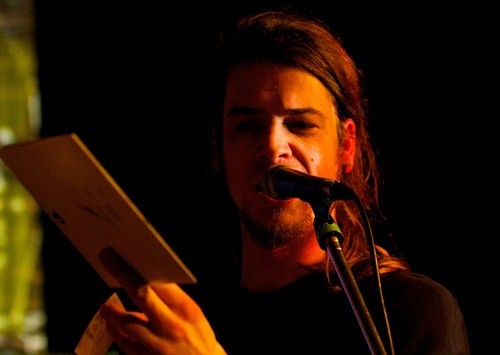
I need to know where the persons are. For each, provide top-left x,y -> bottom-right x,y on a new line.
98,12 -> 470,355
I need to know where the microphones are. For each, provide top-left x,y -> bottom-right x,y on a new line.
260,164 -> 353,202
